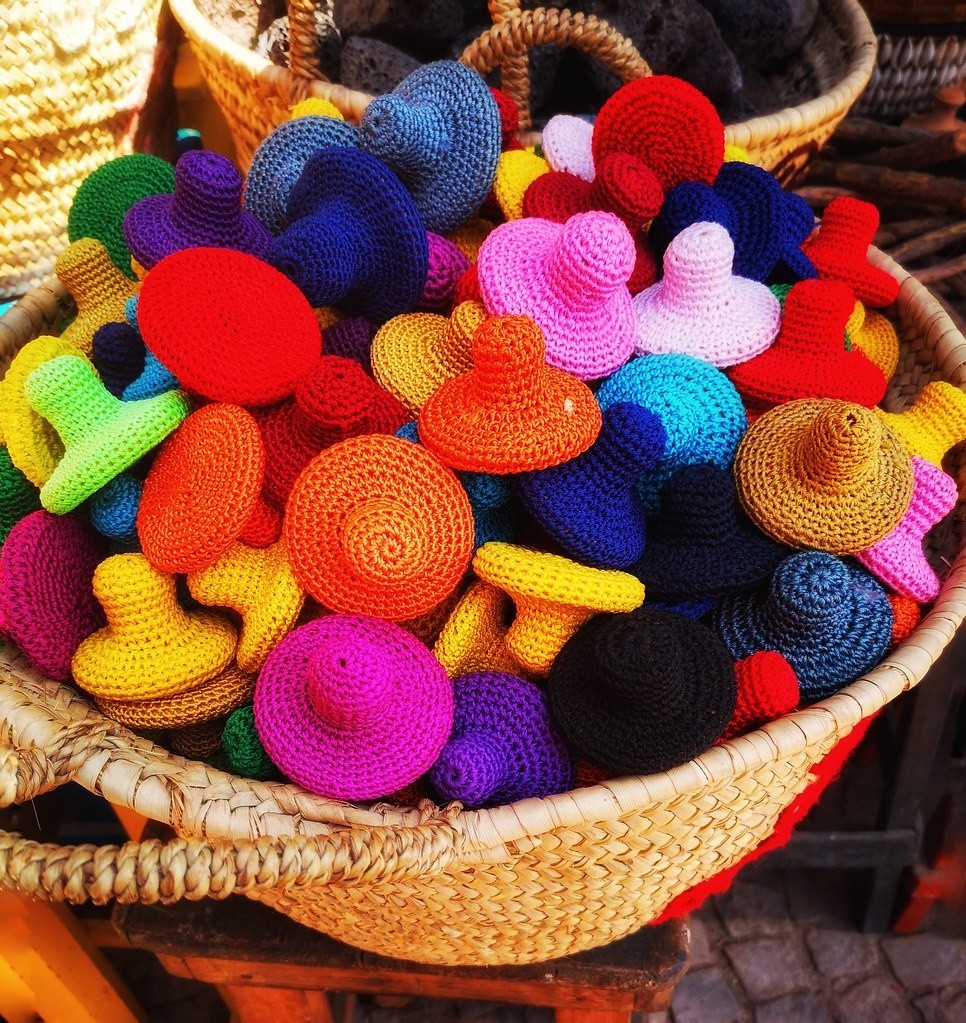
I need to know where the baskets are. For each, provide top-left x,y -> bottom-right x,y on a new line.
0,0 -> 966,968
